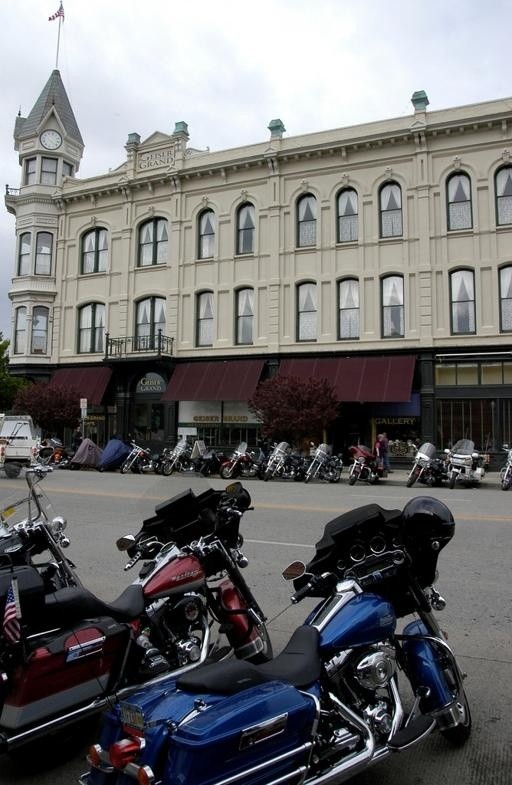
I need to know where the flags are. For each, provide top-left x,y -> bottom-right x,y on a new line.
3,578 -> 23,645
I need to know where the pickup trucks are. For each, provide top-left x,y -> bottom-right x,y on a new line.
0,414 -> 42,479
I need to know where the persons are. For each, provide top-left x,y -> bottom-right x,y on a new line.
72,427 -> 83,447
373,432 -> 384,476
381,432 -> 394,473
134,428 -> 145,442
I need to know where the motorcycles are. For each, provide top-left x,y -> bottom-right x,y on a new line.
500,444 -> 512,491
